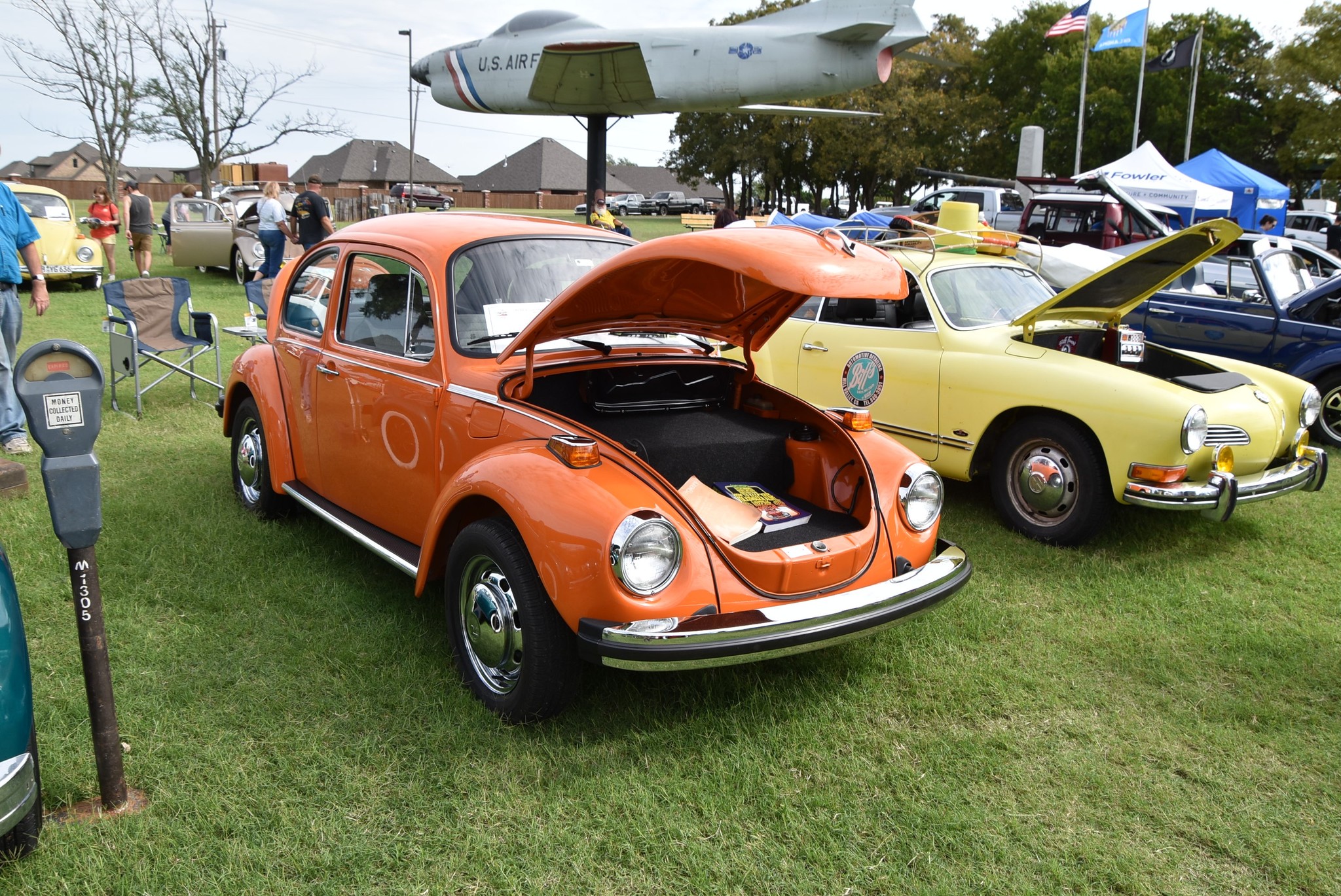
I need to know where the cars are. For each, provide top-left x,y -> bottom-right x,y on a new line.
1101,233 -> 1341,307
216,213 -> 973,730
169,179 -> 338,286
1012,176 -> 1189,250
389,183 -> 455,211
871,186 -> 1091,234
608,218 -> 1329,546
574,196 -> 616,216
1010,229 -> 1341,449
187,183 -> 227,213
1,180 -> 105,292
1283,210 -> 1338,251
605,193 -> 646,216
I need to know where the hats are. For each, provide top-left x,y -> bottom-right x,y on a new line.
124,180 -> 138,190
597,199 -> 605,204
308,175 -> 321,184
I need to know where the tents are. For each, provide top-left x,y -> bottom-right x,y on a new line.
1070,140 -> 1234,226
1161,147 -> 1291,237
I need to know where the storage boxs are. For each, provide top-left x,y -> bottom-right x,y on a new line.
217,161 -> 289,187
899,200 -> 1023,256
1287,198 -> 1337,213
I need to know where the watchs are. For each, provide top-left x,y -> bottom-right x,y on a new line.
32,274 -> 45,280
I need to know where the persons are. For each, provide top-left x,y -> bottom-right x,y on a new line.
756,196 -> 762,216
747,196 -> 755,216
714,208 -> 740,229
590,199 -> 633,238
122,180 -> 154,279
87,186 -> 121,281
1326,214 -> 1341,259
1258,214 -> 1277,234
0,182 -> 51,454
290,175 -> 335,253
252,181 -> 299,281
161,184 -> 197,257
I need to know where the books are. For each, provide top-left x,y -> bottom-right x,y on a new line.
711,481 -> 812,534
678,474 -> 763,546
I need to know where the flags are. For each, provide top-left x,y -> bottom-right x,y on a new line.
1145,34 -> 1196,73
1306,179 -> 1321,197
1044,0 -> 1091,39
1090,8 -> 1148,52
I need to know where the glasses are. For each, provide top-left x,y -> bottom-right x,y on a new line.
93,194 -> 104,199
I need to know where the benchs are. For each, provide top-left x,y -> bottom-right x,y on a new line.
791,298 -> 898,328
744,216 -> 770,226
681,213 -> 740,232
764,214 -> 793,219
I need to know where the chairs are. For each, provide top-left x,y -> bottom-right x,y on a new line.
101,277 -> 225,424
152,222 -> 168,255
836,298 -> 893,328
351,274 -> 435,354
1160,274 -> 1192,293
1181,262 -> 1218,295
898,289 -> 936,331
221,277 -> 276,347
1227,247 -> 1252,268
506,265 -> 593,303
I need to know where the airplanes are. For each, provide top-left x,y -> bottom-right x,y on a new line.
414,0 -> 968,118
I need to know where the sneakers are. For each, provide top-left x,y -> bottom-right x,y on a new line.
1,437 -> 33,454
139,270 -> 149,279
107,273 -> 115,281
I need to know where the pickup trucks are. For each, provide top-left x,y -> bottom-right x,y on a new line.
638,191 -> 704,217
759,195 -> 811,216
826,199 -> 871,218
